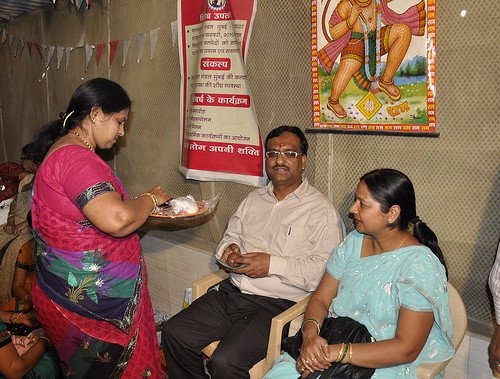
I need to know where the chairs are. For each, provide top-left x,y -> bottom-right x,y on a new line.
265,281 -> 467,379
191,270 -> 305,379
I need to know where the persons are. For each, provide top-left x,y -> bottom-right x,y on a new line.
163,125 -> 346,379
0,142 -> 48,312
487,241 -> 500,374
0,312 -> 53,379
264,168 -> 457,378
22,76 -> 174,379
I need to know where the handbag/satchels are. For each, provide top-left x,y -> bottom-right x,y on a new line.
291,318 -> 376,379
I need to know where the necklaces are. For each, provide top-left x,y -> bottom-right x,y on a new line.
395,234 -> 411,249
68,128 -> 95,153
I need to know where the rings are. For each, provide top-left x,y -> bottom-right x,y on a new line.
298,344 -> 328,372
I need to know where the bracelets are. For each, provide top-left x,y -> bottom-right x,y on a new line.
135,191 -> 159,215
37,336 -> 52,346
12,312 -> 24,324
349,342 -> 353,364
301,318 -> 321,335
9,312 -> 18,324
7,214 -> 15,219
335,343 -> 345,364
339,343 -> 348,364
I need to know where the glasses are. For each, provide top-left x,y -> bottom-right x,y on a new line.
265,150 -> 303,158
